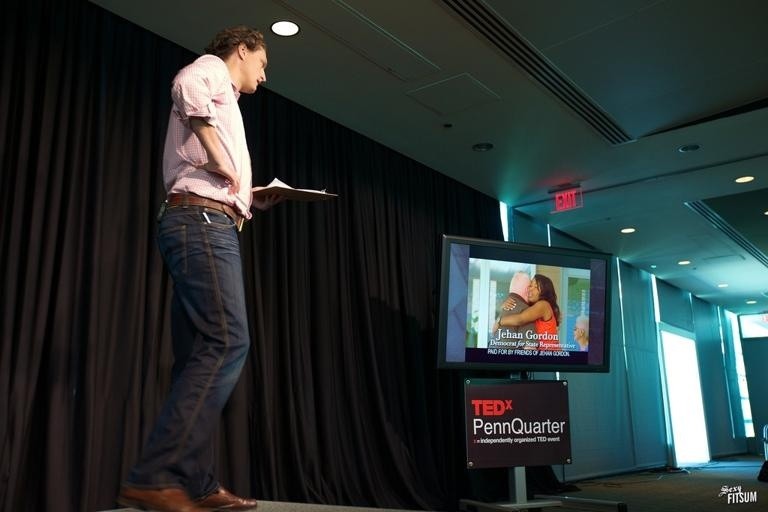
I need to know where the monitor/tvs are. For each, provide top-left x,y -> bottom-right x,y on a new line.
436,233 -> 611,372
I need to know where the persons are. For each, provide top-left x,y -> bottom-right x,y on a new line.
488,272 -> 539,351
116,23 -> 286,511
492,274 -> 562,352
573,316 -> 589,352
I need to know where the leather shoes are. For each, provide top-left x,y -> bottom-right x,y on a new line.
118,485 -> 257,512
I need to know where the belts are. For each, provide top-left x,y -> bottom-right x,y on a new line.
166,193 -> 244,232
